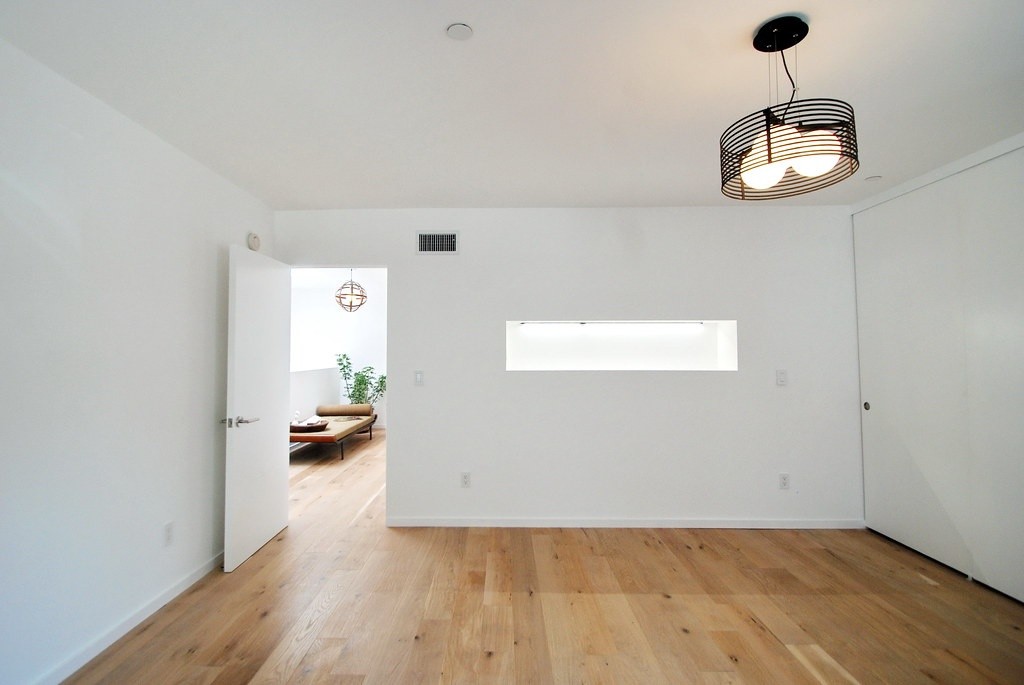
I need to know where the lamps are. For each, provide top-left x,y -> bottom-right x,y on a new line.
720,14 -> 860,202
335,268 -> 368,313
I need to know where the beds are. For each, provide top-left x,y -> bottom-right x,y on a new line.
290,412 -> 378,460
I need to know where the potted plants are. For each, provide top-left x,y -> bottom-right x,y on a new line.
337,353 -> 386,434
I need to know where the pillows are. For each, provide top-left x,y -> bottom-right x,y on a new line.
316,404 -> 374,417
